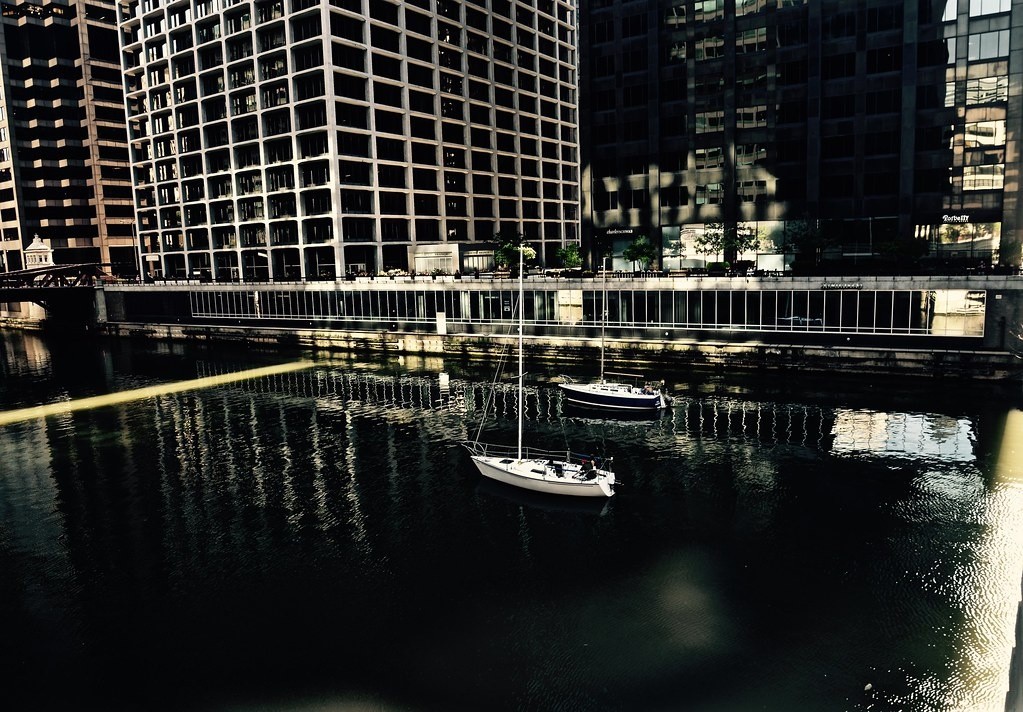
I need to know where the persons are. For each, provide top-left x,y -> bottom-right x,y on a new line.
977,260 -> 985,275
580,460 -> 597,481
434,269 -> 441,279
473,267 -> 479,279
410,269 -> 416,280
369,270 -> 374,280
662,266 -> 671,278
685,268 -> 692,277
455,270 -> 459,279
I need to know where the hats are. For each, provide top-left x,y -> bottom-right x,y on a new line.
580,459 -> 587,464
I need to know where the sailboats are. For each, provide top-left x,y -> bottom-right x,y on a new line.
460,232 -> 623,497
557,251 -> 692,410
